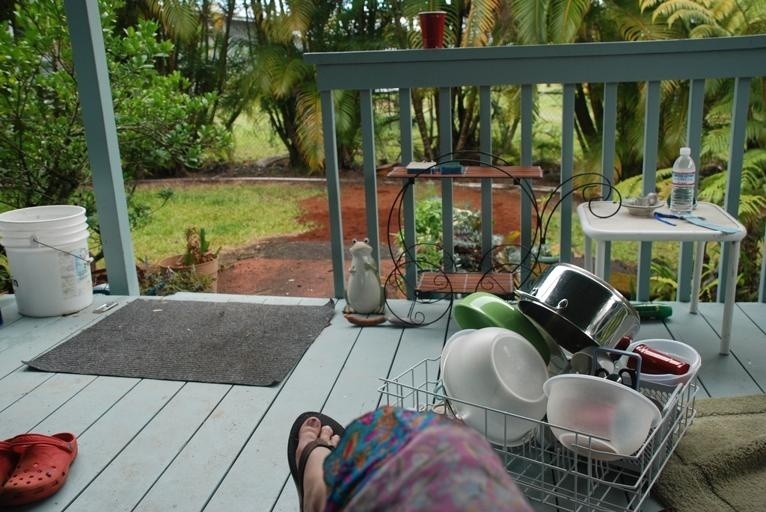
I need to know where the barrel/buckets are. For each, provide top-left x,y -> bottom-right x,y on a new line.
1,203 -> 95,318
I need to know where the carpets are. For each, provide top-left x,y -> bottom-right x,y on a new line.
639,391 -> 766,511
20,296 -> 337,388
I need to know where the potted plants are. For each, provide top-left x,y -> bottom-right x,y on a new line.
158,227 -> 224,294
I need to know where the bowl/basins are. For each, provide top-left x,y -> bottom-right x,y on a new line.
441,327 -> 551,451
617,339 -> 703,389
540,374 -> 663,463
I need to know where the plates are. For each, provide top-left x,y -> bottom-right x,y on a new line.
451,289 -> 572,377
620,199 -> 666,217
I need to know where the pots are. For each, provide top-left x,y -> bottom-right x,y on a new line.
512,261 -> 641,355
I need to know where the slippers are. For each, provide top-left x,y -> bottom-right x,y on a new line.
283,409 -> 347,512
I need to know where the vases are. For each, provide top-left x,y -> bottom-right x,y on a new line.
417,9 -> 448,50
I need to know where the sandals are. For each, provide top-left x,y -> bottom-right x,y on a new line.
0,429 -> 78,505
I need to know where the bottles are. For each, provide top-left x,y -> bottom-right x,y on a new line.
670,147 -> 697,214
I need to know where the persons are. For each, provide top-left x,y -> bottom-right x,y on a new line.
283,402 -> 536,511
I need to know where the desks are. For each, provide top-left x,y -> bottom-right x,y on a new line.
576,198 -> 749,359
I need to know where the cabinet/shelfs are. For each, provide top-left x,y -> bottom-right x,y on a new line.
385,163 -> 546,328
